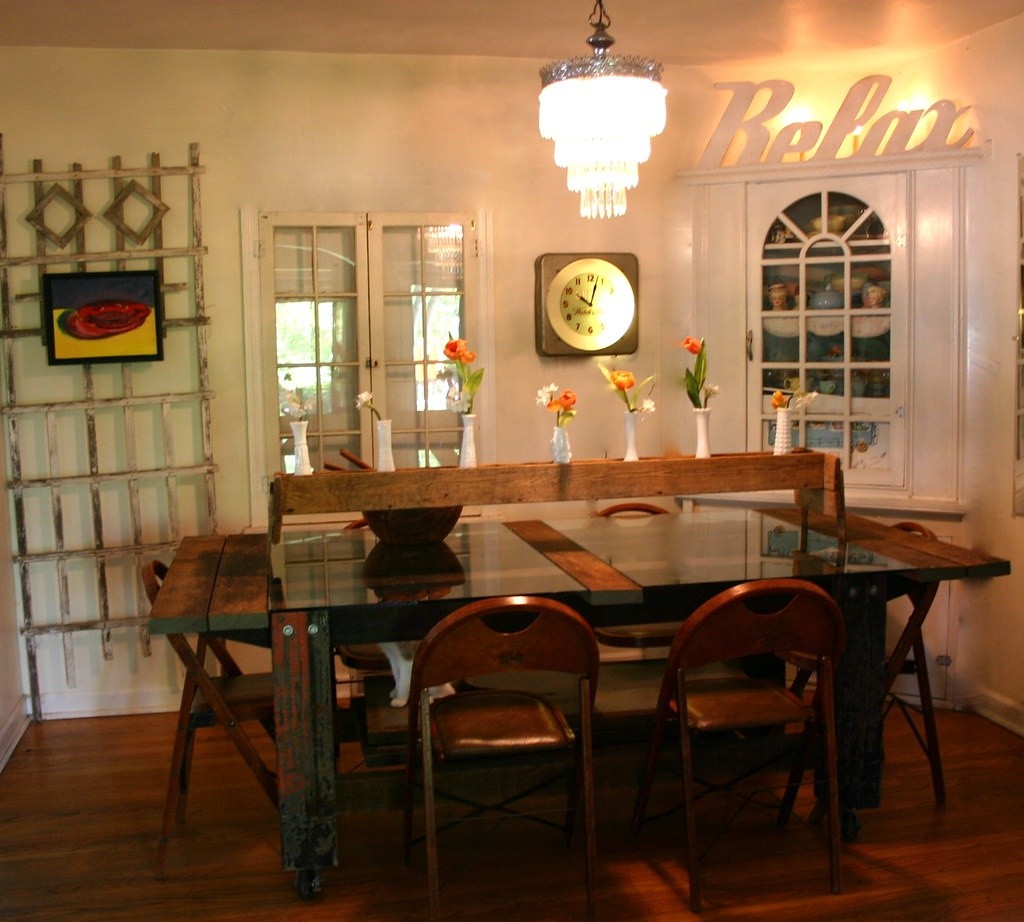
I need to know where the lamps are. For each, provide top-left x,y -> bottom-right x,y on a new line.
538,0 -> 667,220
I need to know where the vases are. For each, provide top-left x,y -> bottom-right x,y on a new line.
459,415 -> 477,467
291,422 -> 313,477
623,413 -> 640,461
693,408 -> 712,459
551,427 -> 572,463
377,420 -> 395,472
773,409 -> 792,456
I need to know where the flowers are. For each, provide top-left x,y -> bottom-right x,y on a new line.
281,389 -> 313,421
536,383 -> 577,426
597,364 -> 657,421
681,335 -> 719,409
436,331 -> 484,414
354,391 -> 381,421
770,378 -> 818,408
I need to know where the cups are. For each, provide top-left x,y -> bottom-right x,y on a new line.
819,380 -> 835,393
784,377 -> 799,391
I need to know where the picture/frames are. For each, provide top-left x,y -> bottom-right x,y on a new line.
42,270 -> 162,366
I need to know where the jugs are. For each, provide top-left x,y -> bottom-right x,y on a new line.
805,283 -> 842,308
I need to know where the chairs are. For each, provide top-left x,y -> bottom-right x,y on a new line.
595,503 -> 683,647
402,596 -> 600,922
777,523 -> 944,826
142,560 -> 280,881
630,578 -> 847,914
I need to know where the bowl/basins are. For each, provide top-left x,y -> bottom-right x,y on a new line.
362,542 -> 465,590
362,506 -> 462,546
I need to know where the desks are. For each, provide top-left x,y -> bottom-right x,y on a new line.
148,509 -> 1009,901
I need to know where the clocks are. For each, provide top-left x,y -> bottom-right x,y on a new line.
535,252 -> 640,357
